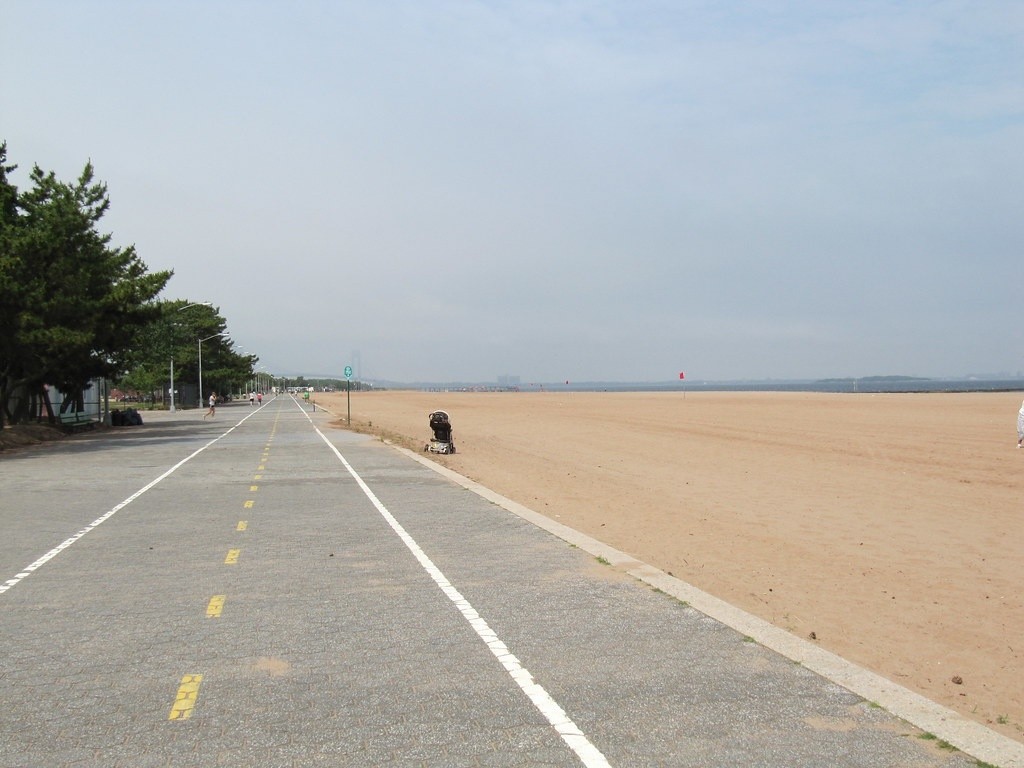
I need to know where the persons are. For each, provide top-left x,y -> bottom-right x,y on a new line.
304,391 -> 309,404
203,392 -> 217,421
249,390 -> 254,407
295,389 -> 297,399
257,391 -> 262,405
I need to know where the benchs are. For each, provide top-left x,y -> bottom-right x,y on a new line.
59,411 -> 100,434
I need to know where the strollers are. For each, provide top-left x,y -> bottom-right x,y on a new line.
423,411 -> 456,454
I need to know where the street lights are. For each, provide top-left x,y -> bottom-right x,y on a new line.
170,302 -> 213,411
245,363 -> 274,395
198,333 -> 230,409
